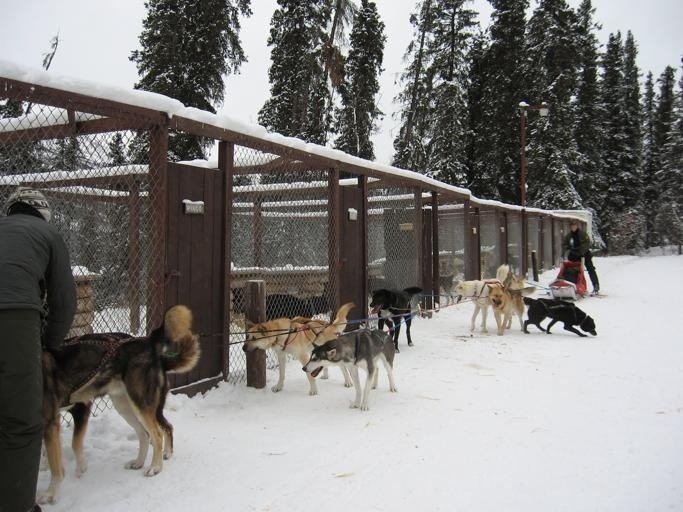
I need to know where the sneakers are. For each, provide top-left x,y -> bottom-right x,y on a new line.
590,289 -> 599,294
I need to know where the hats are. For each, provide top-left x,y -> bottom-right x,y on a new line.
2,185 -> 50,217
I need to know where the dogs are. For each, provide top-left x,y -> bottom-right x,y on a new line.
369,286 -> 423,353
302,329 -> 397,411
36,303 -> 202,506
522,296 -> 597,337
228,287 -> 331,322
439,263 -> 535,336
242,301 -> 357,396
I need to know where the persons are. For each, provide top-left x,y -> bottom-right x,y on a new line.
561,220 -> 600,297
0,186 -> 76,511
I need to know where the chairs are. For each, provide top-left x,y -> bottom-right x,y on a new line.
550,261 -> 586,301
